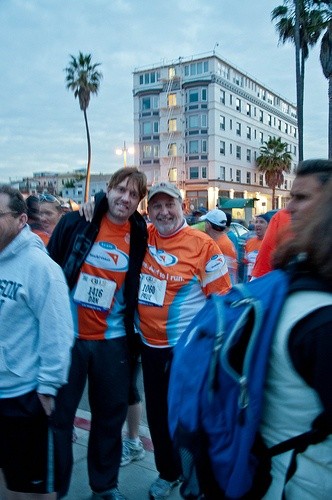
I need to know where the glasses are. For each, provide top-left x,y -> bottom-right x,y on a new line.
39,194 -> 61,205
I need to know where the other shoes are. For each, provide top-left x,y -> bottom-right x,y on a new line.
150,476 -> 178,499
92,489 -> 126,500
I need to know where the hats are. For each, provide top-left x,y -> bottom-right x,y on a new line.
201,209 -> 227,226
256,214 -> 270,223
147,181 -> 181,199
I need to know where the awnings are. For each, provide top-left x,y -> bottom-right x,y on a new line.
217,198 -> 260,208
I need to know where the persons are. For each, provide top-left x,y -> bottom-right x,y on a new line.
192,208 -> 238,285
46,167 -> 149,500
249,208 -> 292,281
28,194 -> 72,246
120,351 -> 145,466
243,214 -> 270,282
286,159 -> 332,218
80,181 -> 234,500
250,178 -> 332,500
0,184 -> 73,494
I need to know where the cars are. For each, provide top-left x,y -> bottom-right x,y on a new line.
257,210 -> 280,223
227,222 -> 260,277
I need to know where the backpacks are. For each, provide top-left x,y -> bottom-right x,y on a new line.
166,265 -> 332,500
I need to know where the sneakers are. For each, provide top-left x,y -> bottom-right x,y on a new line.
120,438 -> 146,466
